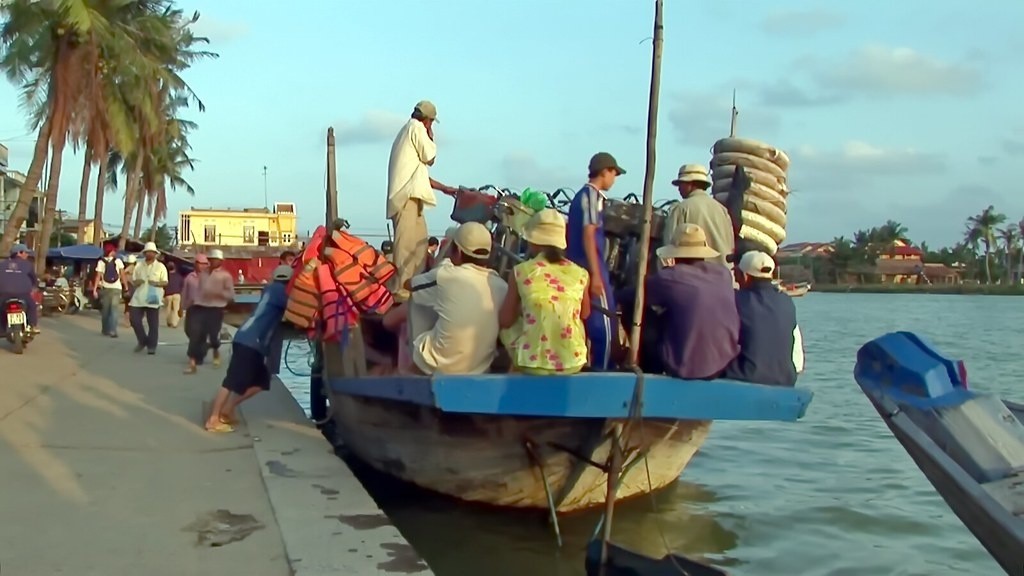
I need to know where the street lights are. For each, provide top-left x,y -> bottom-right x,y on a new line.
262,166 -> 269,209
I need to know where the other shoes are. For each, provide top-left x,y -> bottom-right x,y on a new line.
183,366 -> 196,374
135,344 -> 145,352
213,354 -> 220,367
28,329 -> 41,335
148,347 -> 155,355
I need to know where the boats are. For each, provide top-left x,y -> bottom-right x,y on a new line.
282,0 -> 816,514
770,279 -> 813,297
852,330 -> 1024,576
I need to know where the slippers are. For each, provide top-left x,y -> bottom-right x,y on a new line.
220,416 -> 237,424
207,423 -> 236,433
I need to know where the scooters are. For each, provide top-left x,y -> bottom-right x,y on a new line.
5,297 -> 41,355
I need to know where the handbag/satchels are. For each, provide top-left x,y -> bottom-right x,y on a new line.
145,284 -> 159,305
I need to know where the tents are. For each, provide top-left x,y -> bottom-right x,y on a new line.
27,243 -> 104,279
88,233 -> 197,278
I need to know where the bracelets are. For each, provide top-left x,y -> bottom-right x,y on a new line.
124,289 -> 129,292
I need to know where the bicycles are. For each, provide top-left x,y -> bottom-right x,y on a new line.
42,283 -> 82,316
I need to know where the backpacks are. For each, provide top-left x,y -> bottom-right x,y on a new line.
100,257 -> 119,283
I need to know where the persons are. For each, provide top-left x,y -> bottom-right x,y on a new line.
301,242 -> 307,251
93,244 -> 130,338
497,211 -> 591,377
183,249 -> 236,375
719,251 -> 798,389
265,251 -> 297,376
178,253 -> 213,365
427,236 -> 439,258
164,261 -> 184,328
726,246 -> 804,373
566,153 -> 621,372
121,255 -> 138,314
128,244 -> 168,355
386,101 -> 440,298
613,225 -> 743,379
402,221 -> 508,376
0,245 -> 41,337
663,164 -> 740,292
204,265 -> 294,433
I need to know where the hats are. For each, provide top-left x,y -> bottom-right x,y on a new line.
140,241 -> 161,256
671,164 -> 713,186
194,254 -> 209,264
590,153 -> 626,175
415,101 -> 440,124
656,223 -> 721,258
206,249 -> 225,261
104,244 -> 117,251
125,254 -> 136,263
10,244 -> 32,255
524,209 -> 568,251
726,241 -> 770,265
738,250 -> 775,280
444,222 -> 491,259
272,265 -> 294,281
